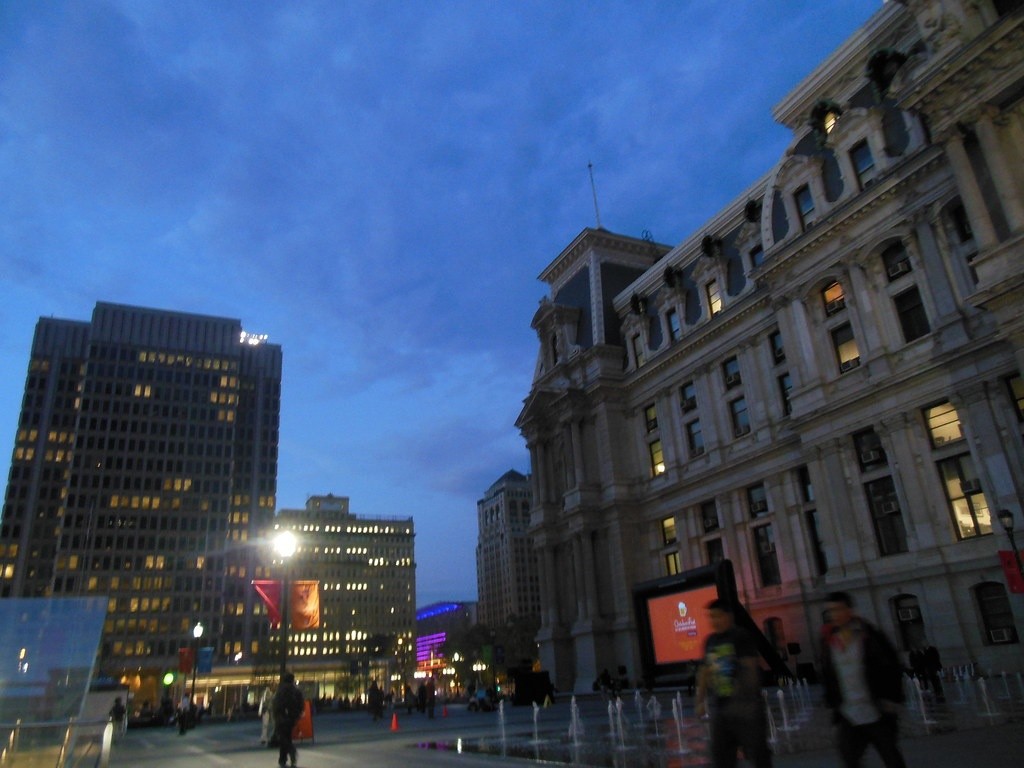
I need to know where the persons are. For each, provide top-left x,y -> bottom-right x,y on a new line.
109,697 -> 127,741
696,598 -> 779,768
258,672 -> 306,767
911,636 -> 944,688
140,691 -> 193,736
598,669 -> 656,704
814,591 -> 910,767
321,677 -> 436,722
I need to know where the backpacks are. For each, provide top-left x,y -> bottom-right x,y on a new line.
283,682 -> 304,718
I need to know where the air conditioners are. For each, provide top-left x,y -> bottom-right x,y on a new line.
647,420 -> 656,429
787,404 -> 792,413
680,400 -> 691,409
805,220 -> 813,229
841,360 -> 858,372
751,500 -> 766,512
692,446 -> 704,457
726,373 -> 740,385
776,346 -> 784,358
960,478 -> 981,495
703,518 -> 718,528
826,300 -> 843,313
881,501 -> 899,513
864,178 -> 877,189
899,608 -> 919,621
735,425 -> 749,437
965,223 -> 973,234
990,628 -> 1015,643
888,263 -> 909,278
861,450 -> 880,463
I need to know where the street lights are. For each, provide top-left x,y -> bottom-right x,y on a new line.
186,622 -> 205,728
276,530 -> 298,676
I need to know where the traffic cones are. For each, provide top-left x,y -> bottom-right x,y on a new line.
390,712 -> 398,730
443,703 -> 449,718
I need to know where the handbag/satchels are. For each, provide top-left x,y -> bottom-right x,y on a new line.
592,681 -> 602,691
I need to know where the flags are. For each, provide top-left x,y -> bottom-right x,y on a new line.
178,647 -> 215,673
